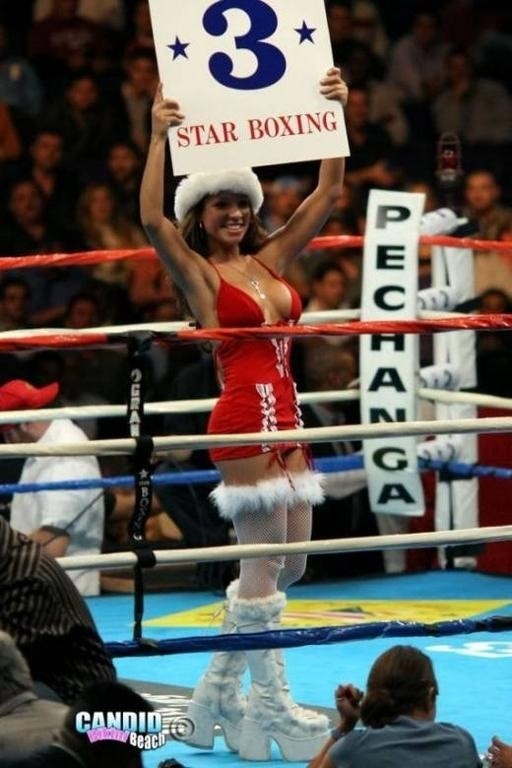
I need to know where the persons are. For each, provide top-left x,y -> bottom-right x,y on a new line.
487,737 -> 511,768
309,647 -> 480,768
2,515 -> 117,703
20,685 -> 156,765
0,0 -> 511,597
141,68 -> 349,762
0,632 -> 72,762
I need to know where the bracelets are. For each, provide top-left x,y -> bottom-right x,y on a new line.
330,728 -> 346,737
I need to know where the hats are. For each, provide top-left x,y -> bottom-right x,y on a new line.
0,380 -> 60,411
173,166 -> 264,220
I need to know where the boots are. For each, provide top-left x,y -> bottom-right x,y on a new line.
233,592 -> 331,764
185,579 -> 249,752
